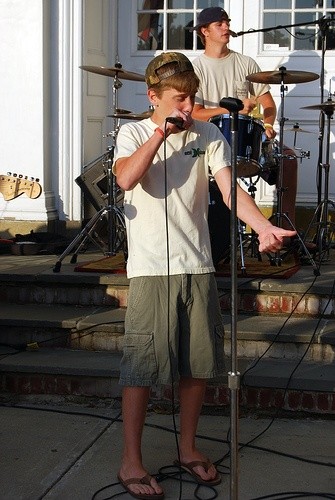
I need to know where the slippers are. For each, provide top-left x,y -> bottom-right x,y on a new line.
173,457 -> 222,486
117,471 -> 164,500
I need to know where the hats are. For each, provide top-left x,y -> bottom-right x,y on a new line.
145,52 -> 194,90
189,7 -> 231,32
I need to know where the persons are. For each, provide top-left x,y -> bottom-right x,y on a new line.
184,6 -> 298,267
112,52 -> 298,500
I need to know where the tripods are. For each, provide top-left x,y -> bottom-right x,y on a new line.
53,73 -> 128,272
237,77 -> 335,276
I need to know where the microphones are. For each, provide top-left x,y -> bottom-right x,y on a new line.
229,30 -> 237,37
166,117 -> 185,129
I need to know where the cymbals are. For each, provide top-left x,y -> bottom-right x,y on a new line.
283,123 -> 322,136
245,66 -> 319,87
107,110 -> 144,121
78,63 -> 147,83
298,96 -> 334,113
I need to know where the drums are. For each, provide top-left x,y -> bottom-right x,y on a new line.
208,112 -> 267,177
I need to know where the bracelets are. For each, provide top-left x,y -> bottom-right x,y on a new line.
154,127 -> 166,140
263,123 -> 273,129
